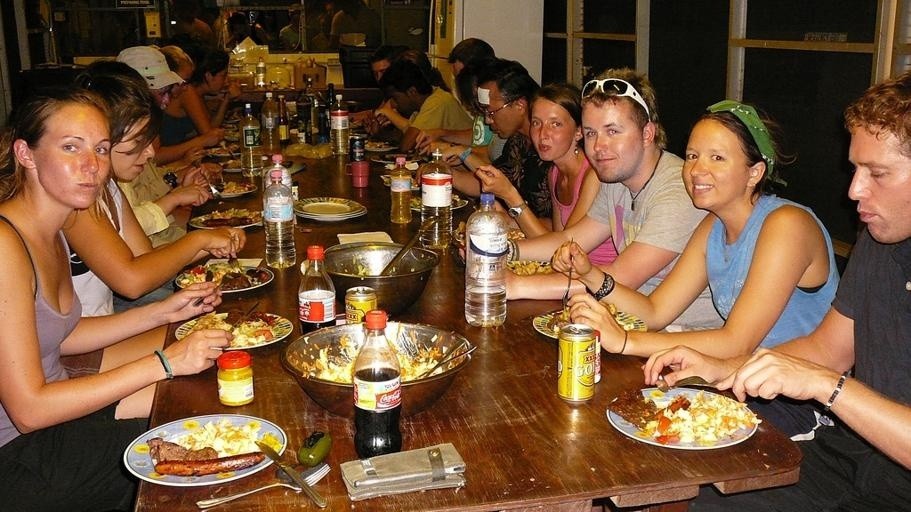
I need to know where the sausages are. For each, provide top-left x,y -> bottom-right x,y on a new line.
154,451 -> 265,476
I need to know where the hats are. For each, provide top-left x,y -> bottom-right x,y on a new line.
118,46 -> 186,86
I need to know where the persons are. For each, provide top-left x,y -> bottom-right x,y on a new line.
61,45 -> 244,378
415,59 -> 556,217
474,82 -> 618,265
549,100 -> 847,443
1,70 -> 235,510
366,39 -> 508,165
176,0 -> 381,51
639,71 -> 911,510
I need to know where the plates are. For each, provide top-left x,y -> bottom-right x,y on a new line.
606,383 -> 758,454
189,208 -> 261,231
217,160 -> 242,173
532,306 -> 647,347
204,146 -> 242,155
172,308 -> 293,351
205,181 -> 258,197
293,196 -> 368,222
175,262 -> 276,294
121,413 -> 289,488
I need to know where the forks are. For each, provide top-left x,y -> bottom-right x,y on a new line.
194,462 -> 335,508
561,233 -> 577,320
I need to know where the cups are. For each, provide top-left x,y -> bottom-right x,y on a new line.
344,161 -> 369,188
349,136 -> 365,163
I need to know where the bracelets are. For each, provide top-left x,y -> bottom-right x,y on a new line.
507,238 -> 519,261
827,372 -> 847,408
621,328 -> 632,356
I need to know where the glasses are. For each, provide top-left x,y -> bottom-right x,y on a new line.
580,79 -> 652,122
477,98 -> 518,115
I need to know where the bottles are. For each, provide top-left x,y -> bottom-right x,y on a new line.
351,307 -> 401,460
298,242 -> 339,337
420,147 -> 453,250
262,92 -> 278,148
262,154 -> 293,191
262,170 -> 296,269
389,156 -> 413,224
277,95 -> 290,149
330,94 -> 350,157
596,327 -> 603,384
462,193 -> 510,327
239,108 -> 263,178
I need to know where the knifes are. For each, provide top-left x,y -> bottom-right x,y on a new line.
249,437 -> 330,512
648,372 -> 672,396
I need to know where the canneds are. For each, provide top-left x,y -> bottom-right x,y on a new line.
558,324 -> 596,403
350,137 -> 364,162
594,330 -> 601,383
345,286 -> 378,325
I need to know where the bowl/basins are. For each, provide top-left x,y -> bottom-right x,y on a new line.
281,320 -> 478,420
320,242 -> 441,313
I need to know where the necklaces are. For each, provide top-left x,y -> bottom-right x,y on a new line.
630,149 -> 664,210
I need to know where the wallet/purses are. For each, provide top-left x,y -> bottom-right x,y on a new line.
339,443 -> 467,501
